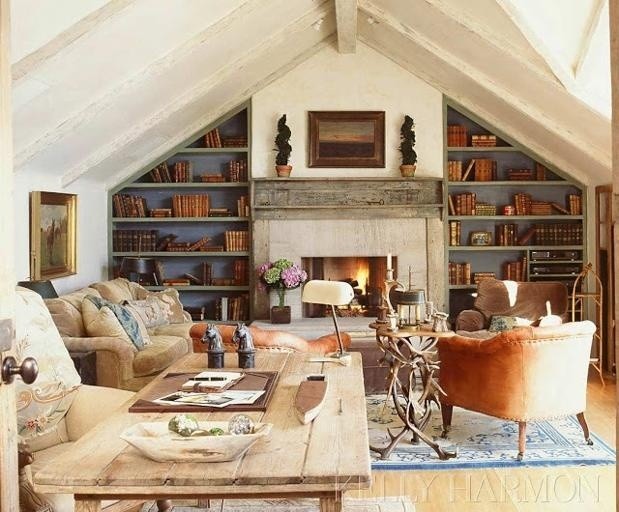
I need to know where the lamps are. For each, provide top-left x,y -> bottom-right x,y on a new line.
300,279 -> 356,359
121,257 -> 154,285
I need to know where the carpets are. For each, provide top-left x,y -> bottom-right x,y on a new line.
366,392 -> 618,469
138,496 -> 417,512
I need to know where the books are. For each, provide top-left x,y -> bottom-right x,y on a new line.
446,123 -> 583,285
183,370 -> 245,391
112,128 -> 248,320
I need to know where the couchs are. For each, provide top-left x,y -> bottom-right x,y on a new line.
17,286 -> 137,512
455,278 -> 569,340
438,321 -> 597,453
190,323 -> 350,356
42,278 -> 193,392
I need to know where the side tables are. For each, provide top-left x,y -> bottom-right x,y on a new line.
368,319 -> 458,461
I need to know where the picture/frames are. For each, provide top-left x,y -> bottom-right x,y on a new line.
28,191 -> 78,281
307,110 -> 385,168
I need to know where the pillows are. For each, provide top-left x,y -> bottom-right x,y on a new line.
134,287 -> 190,325
83,295 -> 152,350
123,296 -> 170,329
489,315 -> 513,333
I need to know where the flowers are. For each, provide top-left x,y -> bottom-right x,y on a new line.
253,259 -> 307,307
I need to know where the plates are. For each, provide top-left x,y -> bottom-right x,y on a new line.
119,421 -> 274,463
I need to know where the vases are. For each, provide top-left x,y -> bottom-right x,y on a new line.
271,306 -> 291,324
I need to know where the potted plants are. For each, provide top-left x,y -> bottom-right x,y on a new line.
272,114 -> 292,177
396,115 -> 417,177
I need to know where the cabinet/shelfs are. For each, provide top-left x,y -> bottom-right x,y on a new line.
571,263 -> 606,388
108,99 -> 250,325
442,95 -> 587,330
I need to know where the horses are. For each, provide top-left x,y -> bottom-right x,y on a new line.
200,323 -> 223,349
231,322 -> 254,350
